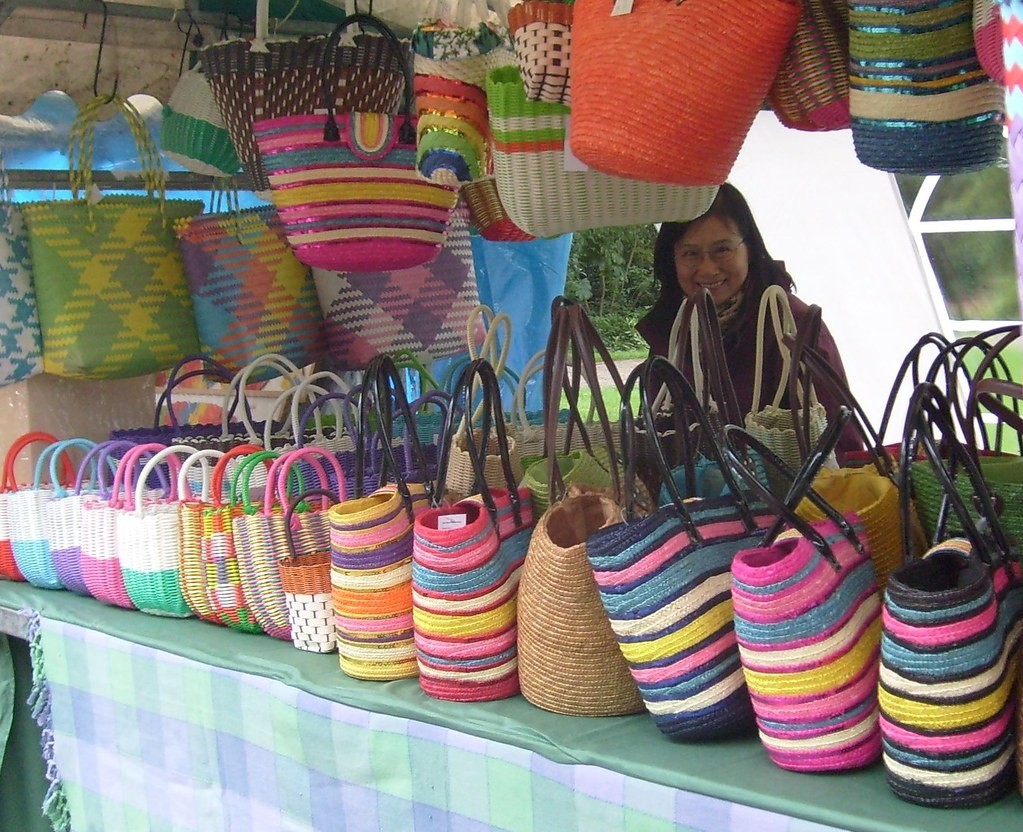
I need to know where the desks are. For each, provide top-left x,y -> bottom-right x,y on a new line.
0,575 -> 1023,832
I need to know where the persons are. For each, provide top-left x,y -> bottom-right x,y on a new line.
633,181 -> 864,503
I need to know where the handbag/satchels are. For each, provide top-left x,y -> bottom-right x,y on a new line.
0,0 -> 1023,808
